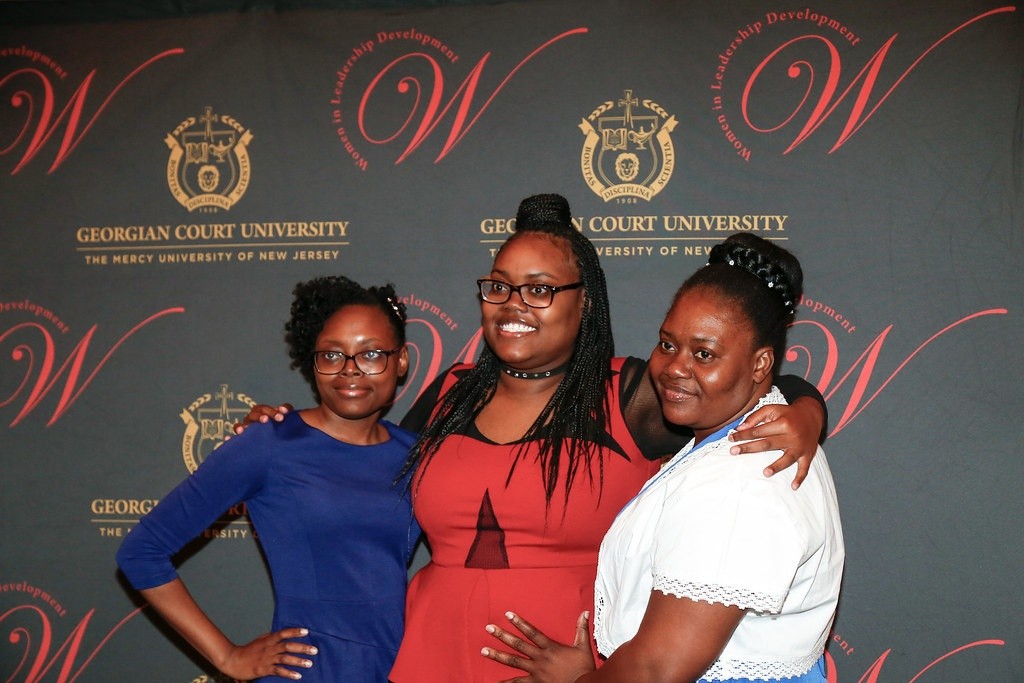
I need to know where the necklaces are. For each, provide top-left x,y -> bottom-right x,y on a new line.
500,360 -> 571,378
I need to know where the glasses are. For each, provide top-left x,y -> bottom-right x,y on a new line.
477,279 -> 584,308
311,346 -> 403,375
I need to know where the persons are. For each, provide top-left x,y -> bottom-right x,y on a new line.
224,193 -> 829,683
481,232 -> 844,683
115,275 -> 425,683
